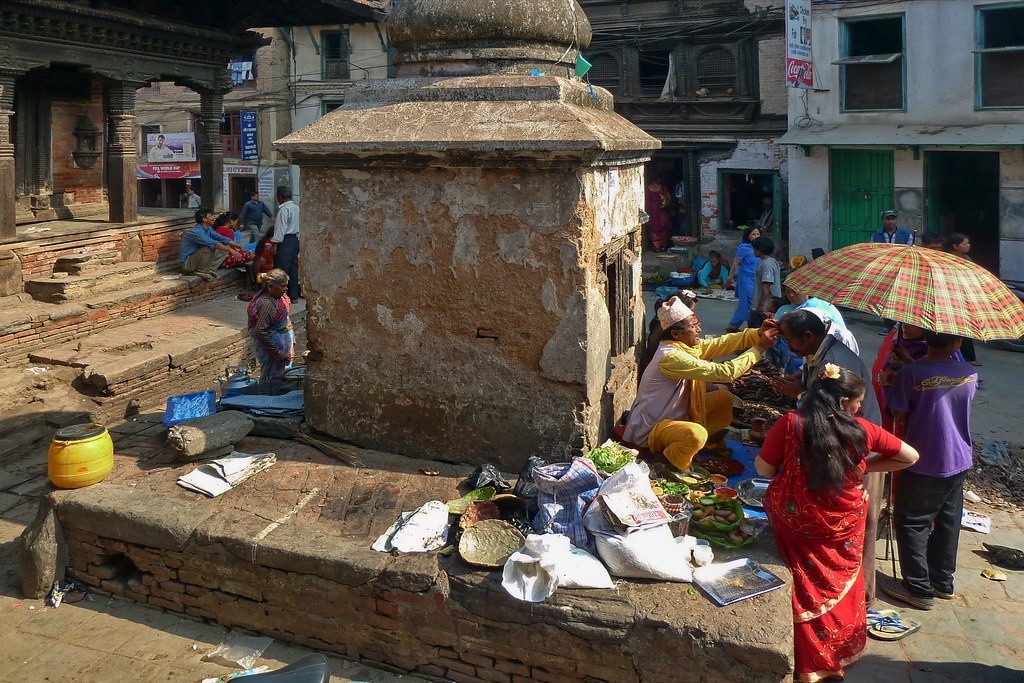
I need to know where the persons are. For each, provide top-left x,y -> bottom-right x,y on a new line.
677,183 -> 683,195
881,330 -> 979,611
622,290 -> 780,485
754,363 -> 919,683
180,185 -> 300,304
645,173 -> 671,252
247,268 -> 296,383
149,134 -> 179,162
726,210 -> 985,539
696,250 -> 729,292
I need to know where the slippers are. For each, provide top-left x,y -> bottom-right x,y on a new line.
869,618 -> 921,640
237,293 -> 254,302
866,607 -> 901,626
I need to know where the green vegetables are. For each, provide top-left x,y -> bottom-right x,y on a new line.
659,481 -> 689,495
582,439 -> 634,473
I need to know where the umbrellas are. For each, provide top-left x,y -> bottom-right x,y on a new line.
784,230 -> 1024,578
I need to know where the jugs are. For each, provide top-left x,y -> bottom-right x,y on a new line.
217,365 -> 258,397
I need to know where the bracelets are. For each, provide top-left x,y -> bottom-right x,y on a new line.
277,351 -> 281,354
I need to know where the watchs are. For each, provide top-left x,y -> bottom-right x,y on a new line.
797,390 -> 808,401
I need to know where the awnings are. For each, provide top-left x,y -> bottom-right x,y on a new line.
776,123 -> 1024,144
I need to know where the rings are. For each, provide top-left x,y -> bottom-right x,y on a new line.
781,386 -> 783,391
774,335 -> 778,340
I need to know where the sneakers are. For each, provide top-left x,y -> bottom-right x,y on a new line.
880,577 -> 935,610
934,588 -> 955,599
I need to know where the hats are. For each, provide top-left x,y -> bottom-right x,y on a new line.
881,209 -> 898,218
657,296 -> 694,331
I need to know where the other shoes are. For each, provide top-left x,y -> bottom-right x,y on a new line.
193,271 -> 213,281
726,324 -> 742,333
879,327 -> 891,335
970,361 -> 982,366
208,268 -> 218,278
